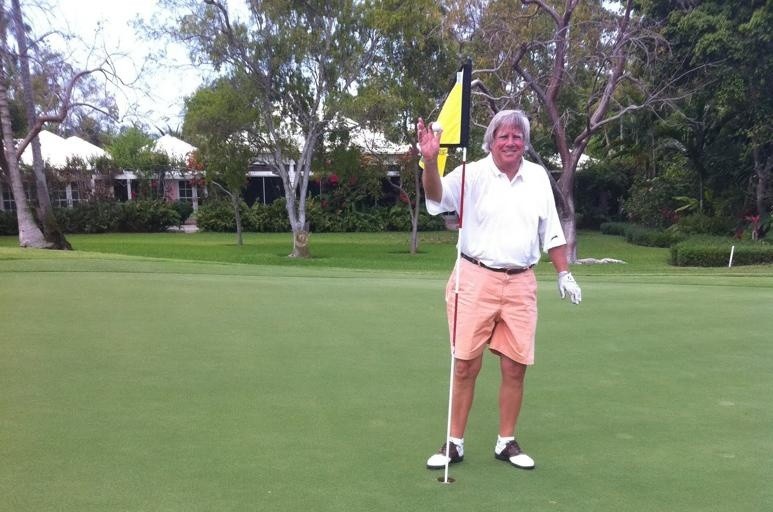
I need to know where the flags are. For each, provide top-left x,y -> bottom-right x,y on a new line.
418,58 -> 472,178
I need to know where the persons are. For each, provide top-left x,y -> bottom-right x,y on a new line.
416,109 -> 582,470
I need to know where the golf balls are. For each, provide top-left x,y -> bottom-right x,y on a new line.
430,120 -> 441,131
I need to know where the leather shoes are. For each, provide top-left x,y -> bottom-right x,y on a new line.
426,437 -> 465,468
494,434 -> 535,469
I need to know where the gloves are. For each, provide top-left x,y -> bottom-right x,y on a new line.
558,271 -> 581,304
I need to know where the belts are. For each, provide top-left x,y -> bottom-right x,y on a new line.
461,252 -> 535,274
